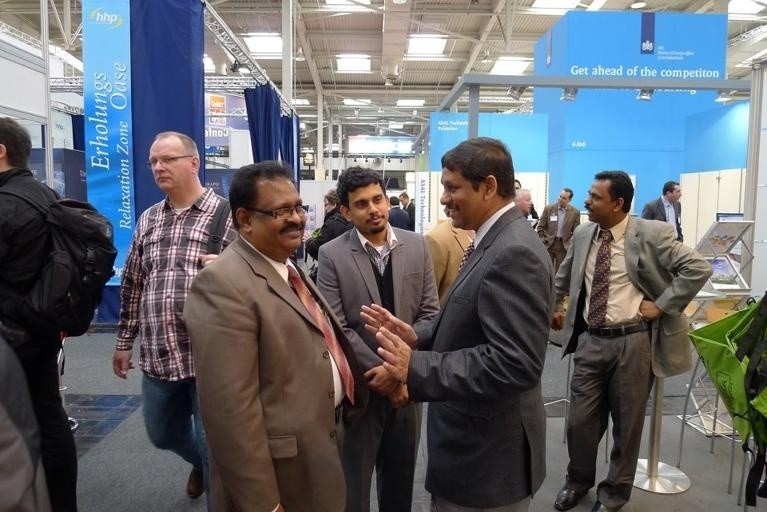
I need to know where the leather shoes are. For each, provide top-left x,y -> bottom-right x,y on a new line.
591,500 -> 619,512
186,469 -> 205,499
554,486 -> 583,511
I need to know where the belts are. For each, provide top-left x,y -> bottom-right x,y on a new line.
584,322 -> 649,339
554,237 -> 562,241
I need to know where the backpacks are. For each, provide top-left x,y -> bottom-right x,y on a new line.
0,181 -> 117,336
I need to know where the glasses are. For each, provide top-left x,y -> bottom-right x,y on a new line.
144,154 -> 196,168
247,204 -> 311,221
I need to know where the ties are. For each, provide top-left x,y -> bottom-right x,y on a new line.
287,265 -> 356,404
457,240 -> 474,270
587,231 -> 612,330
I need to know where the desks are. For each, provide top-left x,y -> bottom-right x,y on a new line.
544,318 -> 610,463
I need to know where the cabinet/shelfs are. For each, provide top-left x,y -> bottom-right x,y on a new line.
686,220 -> 754,330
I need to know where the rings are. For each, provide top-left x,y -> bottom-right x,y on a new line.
379,322 -> 382,329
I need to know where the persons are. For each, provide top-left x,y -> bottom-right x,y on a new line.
642,181 -> 683,243
423,179 -> 581,330
554,171 -> 713,512
0,117 -> 77,512
301,188 -> 415,261
184,160 -> 370,512
317,166 -> 442,512
112,131 -> 240,512
0,320 -> 52,512
360,138 -> 557,512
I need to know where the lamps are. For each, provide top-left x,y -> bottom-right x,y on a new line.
560,87 -> 577,102
636,89 -> 653,101
230,60 -> 239,72
714,89 -> 733,103
506,84 -> 526,100
